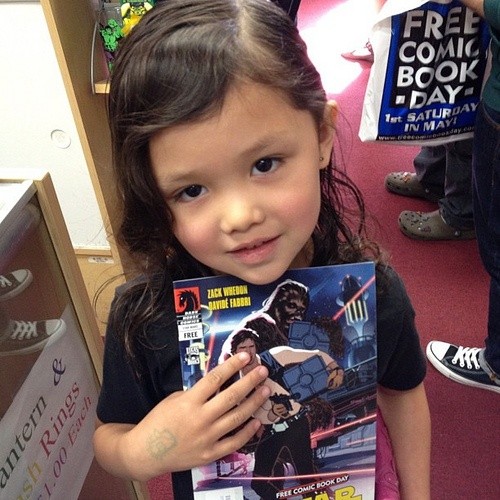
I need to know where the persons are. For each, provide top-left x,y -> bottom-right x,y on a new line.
424,0 -> 500,394
0,269 -> 67,362
94,0 -> 433,500
383,137 -> 478,240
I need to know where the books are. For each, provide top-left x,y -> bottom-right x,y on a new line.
171,261 -> 376,500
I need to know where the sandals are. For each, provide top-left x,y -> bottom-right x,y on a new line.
384,172 -> 433,199
398,209 -> 476,241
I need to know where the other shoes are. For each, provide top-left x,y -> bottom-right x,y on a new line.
1,317 -> 65,358
0,268 -> 33,302
427,339 -> 500,394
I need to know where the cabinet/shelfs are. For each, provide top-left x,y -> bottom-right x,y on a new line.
0,169 -> 151,500
